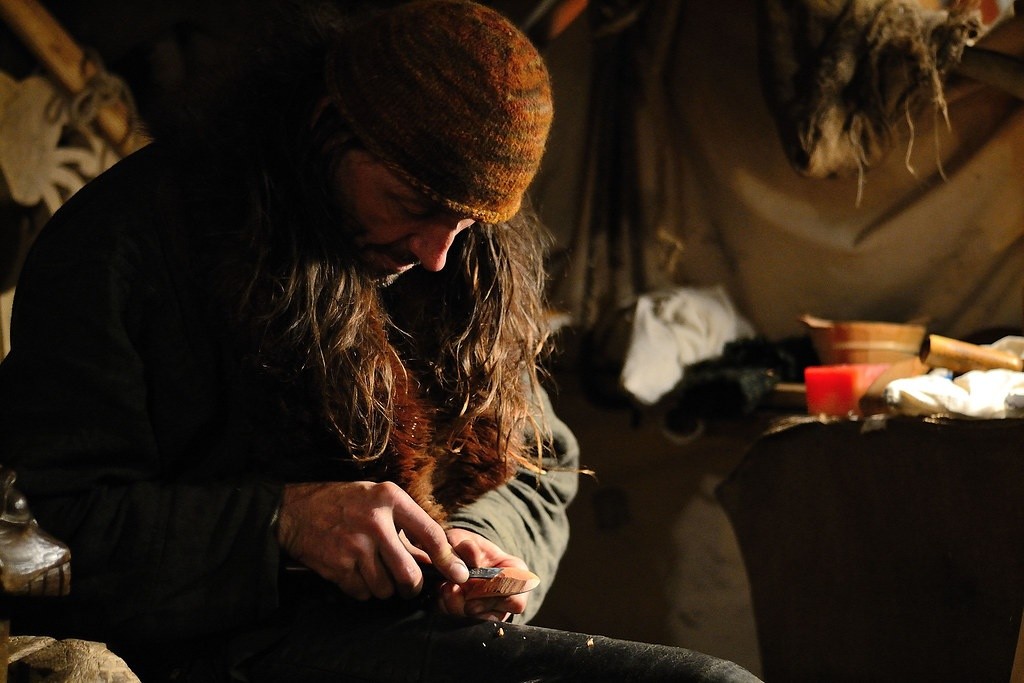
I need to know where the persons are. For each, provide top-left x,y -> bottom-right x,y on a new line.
7,0 -> 581,683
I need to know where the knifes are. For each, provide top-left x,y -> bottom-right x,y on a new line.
468,567 -> 500,579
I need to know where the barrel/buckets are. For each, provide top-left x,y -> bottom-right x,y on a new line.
812,321 -> 925,363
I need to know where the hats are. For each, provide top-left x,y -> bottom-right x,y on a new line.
327,0 -> 553,222
